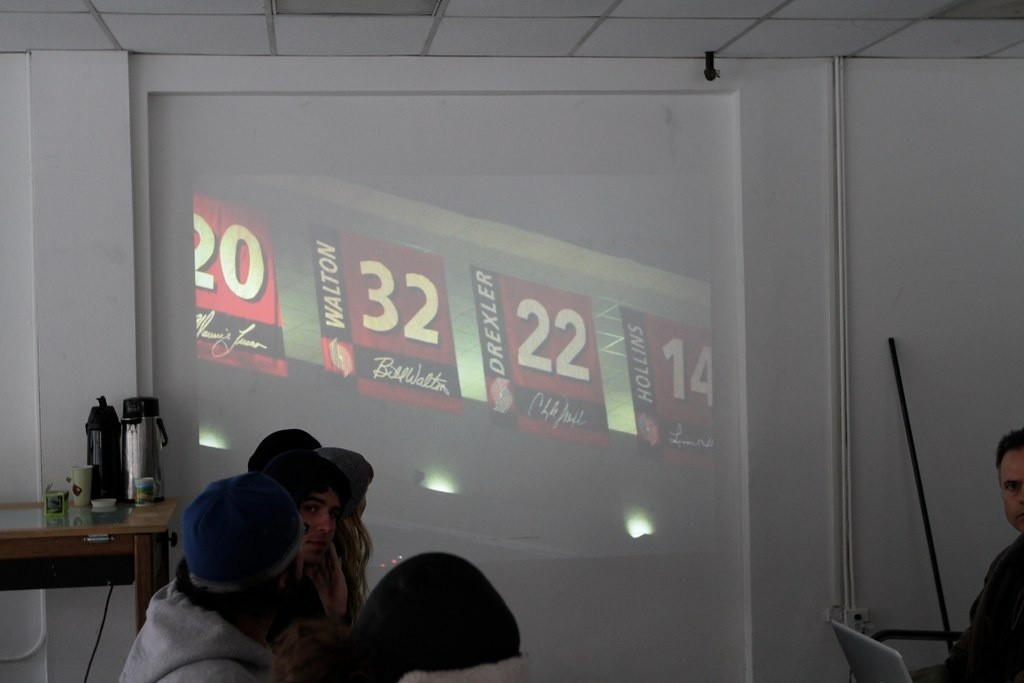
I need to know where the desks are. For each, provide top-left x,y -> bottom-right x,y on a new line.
0,496 -> 179,635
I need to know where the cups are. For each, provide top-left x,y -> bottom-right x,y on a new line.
132,477 -> 161,507
70,465 -> 94,508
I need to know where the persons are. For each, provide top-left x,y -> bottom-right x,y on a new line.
118,472 -> 311,683
321,552 -> 533,683
249,429 -> 375,683
910,428 -> 1024,683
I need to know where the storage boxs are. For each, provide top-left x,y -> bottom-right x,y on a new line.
44,481 -> 70,519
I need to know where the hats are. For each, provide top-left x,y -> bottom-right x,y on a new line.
344,552 -> 520,683
314,446 -> 368,505
247,427 -> 322,471
264,451 -> 351,504
181,473 -> 304,591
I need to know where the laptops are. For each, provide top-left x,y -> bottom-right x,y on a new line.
830,620 -> 913,683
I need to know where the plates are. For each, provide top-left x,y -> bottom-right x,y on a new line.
91,498 -> 117,506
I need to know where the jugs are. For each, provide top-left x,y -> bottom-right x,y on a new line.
121,396 -> 168,503
84,395 -> 121,500
